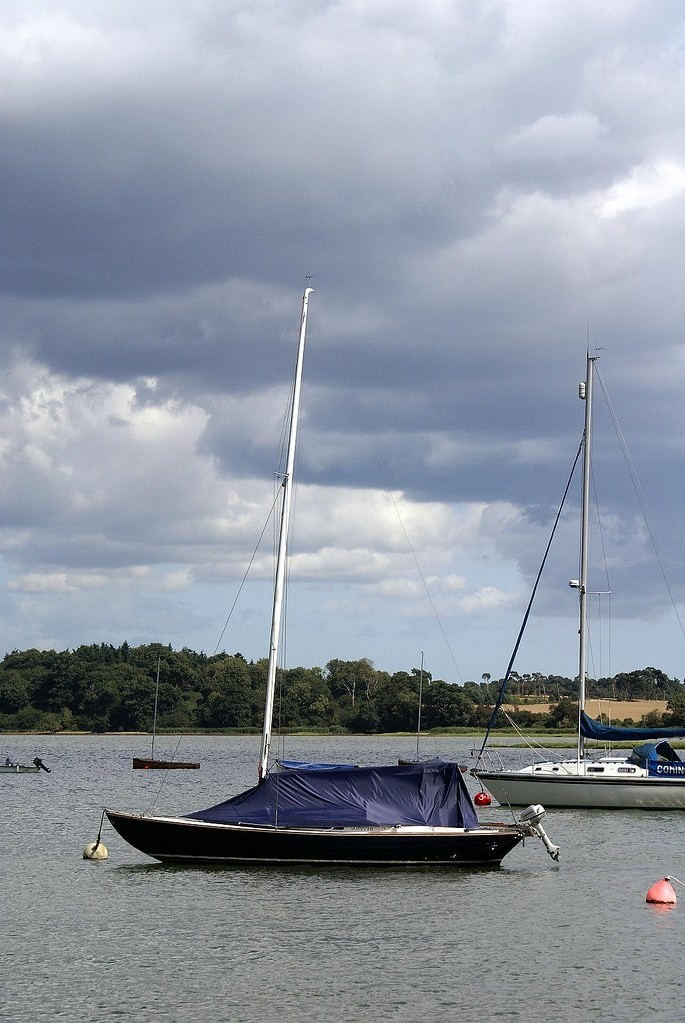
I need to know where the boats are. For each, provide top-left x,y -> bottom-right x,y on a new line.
1,755 -> 52,774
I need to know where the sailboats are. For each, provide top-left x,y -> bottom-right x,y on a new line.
397,649 -> 468,773
133,655 -> 201,770
105,286 -> 560,870
469,320 -> 685,812
